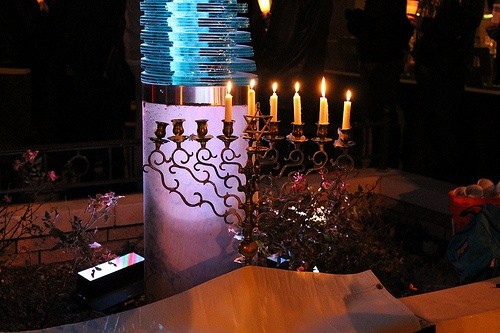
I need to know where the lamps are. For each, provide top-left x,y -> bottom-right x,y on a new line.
405,0 -> 420,22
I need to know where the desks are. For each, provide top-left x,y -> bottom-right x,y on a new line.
20,264 -> 500,333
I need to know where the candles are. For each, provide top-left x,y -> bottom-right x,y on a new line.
342,89 -> 352,130
320,75 -> 329,124
248,78 -> 256,120
224,80 -> 234,122
293,80 -> 302,125
270,81 -> 278,122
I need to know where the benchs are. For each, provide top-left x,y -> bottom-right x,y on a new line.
0,136 -> 144,197
